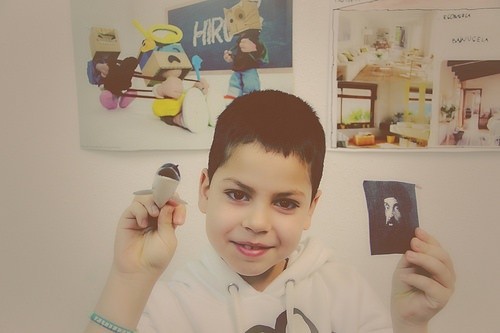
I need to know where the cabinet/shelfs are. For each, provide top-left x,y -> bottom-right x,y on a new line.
336,126 -> 375,145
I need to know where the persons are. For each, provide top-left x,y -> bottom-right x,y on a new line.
224,0 -> 266,103
82,90 -> 455,333
90,26 -> 137,109
364,179 -> 420,255
139,38 -> 222,132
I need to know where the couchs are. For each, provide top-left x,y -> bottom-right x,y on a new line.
336,41 -> 403,81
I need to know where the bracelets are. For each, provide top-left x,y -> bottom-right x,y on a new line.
90,312 -> 135,333
